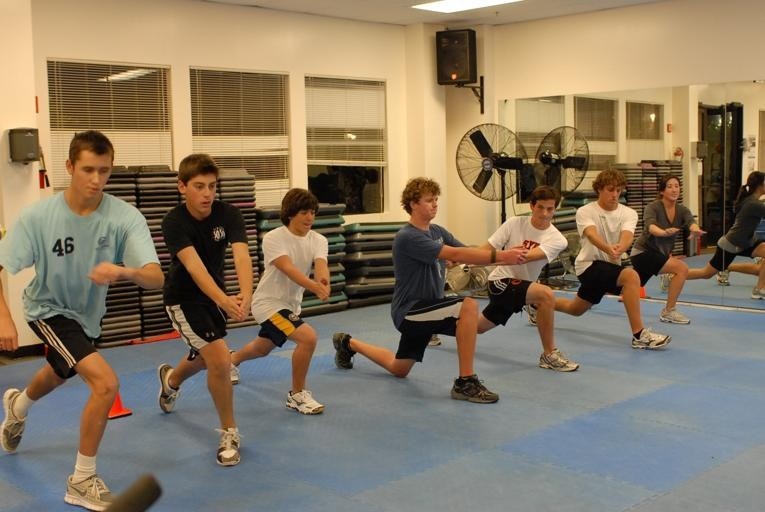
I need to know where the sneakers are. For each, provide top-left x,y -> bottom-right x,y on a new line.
0,388 -> 27,451
523,299 -> 691,372
659,271 -> 765,300
64,474 -> 115,511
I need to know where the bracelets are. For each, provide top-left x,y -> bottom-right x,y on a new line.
490,248 -> 496,264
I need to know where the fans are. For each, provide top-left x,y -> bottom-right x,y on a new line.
533,124 -> 588,289
455,124 -> 528,227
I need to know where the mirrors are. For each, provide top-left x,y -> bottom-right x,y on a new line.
497,79 -> 764,313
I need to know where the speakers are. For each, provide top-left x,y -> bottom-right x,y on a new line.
436,28 -> 477,85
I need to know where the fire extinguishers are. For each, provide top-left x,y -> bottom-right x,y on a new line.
674,147 -> 683,161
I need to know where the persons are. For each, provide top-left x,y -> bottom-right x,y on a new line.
427,185 -> 580,372
228,188 -> 332,416
0,130 -> 166,512
333,177 -> 499,404
629,174 -> 708,324
158,154 -> 254,466
660,171 -> 765,300
522,167 -> 673,350
717,216 -> 765,286
